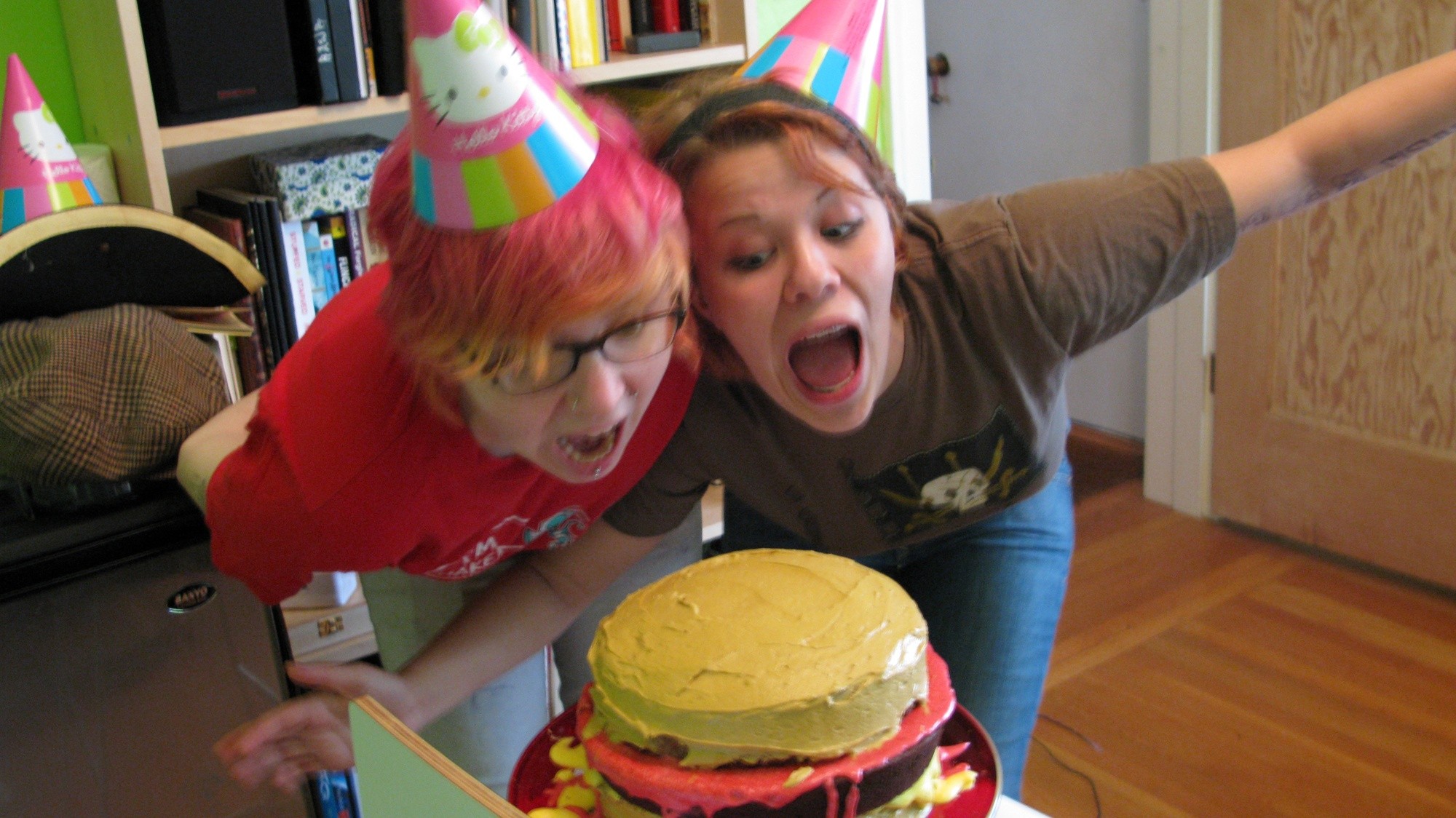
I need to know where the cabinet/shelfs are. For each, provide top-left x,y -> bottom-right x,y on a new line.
60,2 -> 753,397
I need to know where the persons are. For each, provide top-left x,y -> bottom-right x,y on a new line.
215,51 -> 1456,801
207,1 -> 712,792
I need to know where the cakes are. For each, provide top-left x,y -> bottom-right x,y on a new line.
577,546 -> 957,818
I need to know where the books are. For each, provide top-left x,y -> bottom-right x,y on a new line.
488,0 -> 717,74
139,0 -> 412,121
184,188 -> 392,404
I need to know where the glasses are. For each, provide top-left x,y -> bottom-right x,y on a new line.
453,286 -> 690,397
